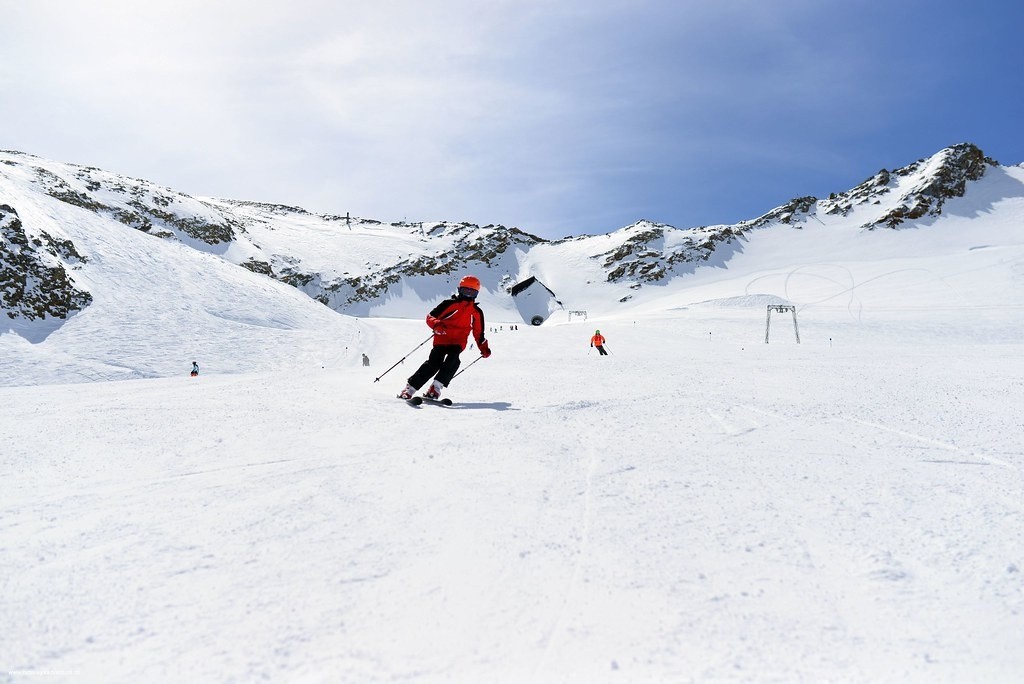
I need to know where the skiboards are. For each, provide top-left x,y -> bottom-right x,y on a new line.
396,393 -> 452,406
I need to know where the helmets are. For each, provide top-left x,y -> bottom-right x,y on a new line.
457,275 -> 481,302
596,330 -> 600,335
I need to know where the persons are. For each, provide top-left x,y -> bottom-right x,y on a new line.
362,353 -> 370,366
399,276 -> 491,399
191,360 -> 199,375
590,330 -> 608,355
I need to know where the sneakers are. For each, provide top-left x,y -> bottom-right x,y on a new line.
399,385 -> 416,399
428,380 -> 444,399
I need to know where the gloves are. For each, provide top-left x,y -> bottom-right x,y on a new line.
434,325 -> 447,336
591,344 -> 593,347
480,349 -> 491,358
603,341 -> 605,343
